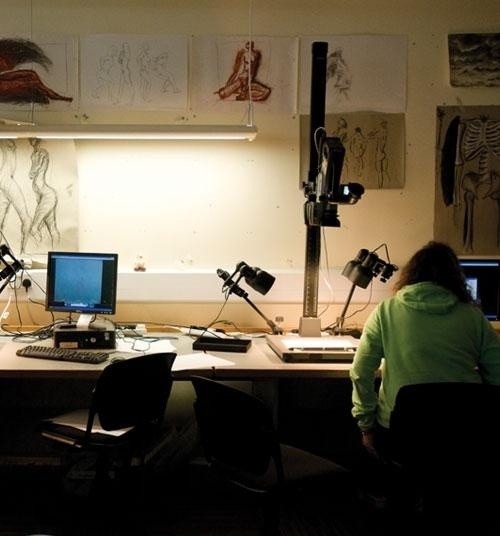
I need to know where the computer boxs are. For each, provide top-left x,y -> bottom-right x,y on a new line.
53,324 -> 115,349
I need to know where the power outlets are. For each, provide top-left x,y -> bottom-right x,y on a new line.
7,272 -> 32,292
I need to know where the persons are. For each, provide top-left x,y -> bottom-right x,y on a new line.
350,241 -> 499,446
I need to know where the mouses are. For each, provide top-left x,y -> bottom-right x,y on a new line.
112,356 -> 124,363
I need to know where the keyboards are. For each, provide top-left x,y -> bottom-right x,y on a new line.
16,345 -> 107,364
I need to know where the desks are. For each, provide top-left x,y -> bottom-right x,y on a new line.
0,328 -> 384,383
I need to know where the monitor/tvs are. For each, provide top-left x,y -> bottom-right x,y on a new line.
457,258 -> 500,322
44,251 -> 118,315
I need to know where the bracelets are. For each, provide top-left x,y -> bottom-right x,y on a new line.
359,429 -> 376,436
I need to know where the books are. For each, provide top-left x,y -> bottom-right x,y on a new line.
192,336 -> 251,353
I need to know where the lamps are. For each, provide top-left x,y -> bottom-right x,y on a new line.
197,260 -> 284,342
323,244 -> 399,339
0,0 -> 257,141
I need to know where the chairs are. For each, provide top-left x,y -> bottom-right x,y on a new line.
41,353 -> 177,501
376,382 -> 500,536
189,375 -> 353,536
